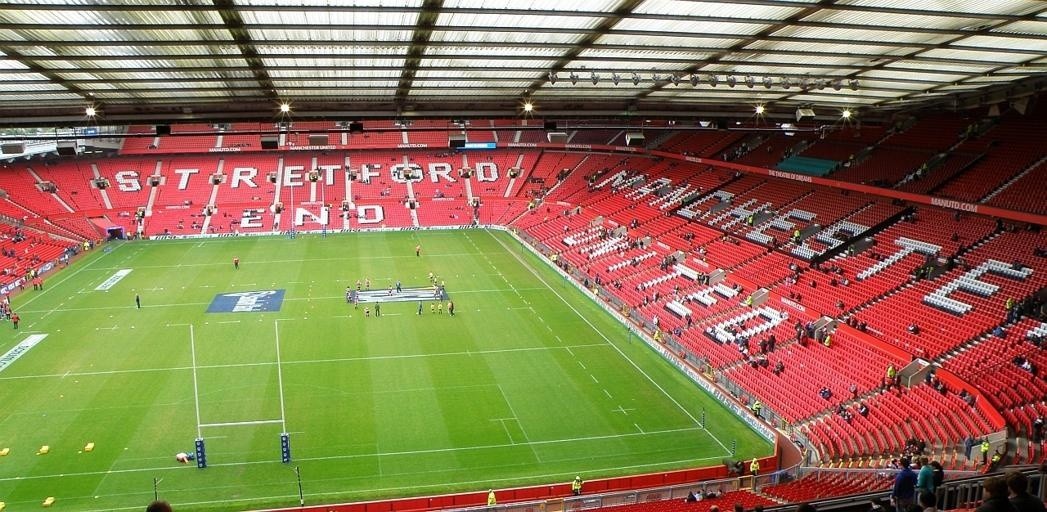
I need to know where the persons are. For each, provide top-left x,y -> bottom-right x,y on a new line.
375,301 -> 381,317
364,277 -> 371,291
343,277 -> 362,310
415,243 -> 421,258
175,452 -> 195,464
135,292 -> 140,309
388,285 -> 393,296
146,499 -> 172,512
364,306 -> 370,317
396,279 -> 402,293
233,256 -> 240,270
428,270 -> 455,316
0,176 -> 112,330
416,300 -> 423,315
487,488 -> 497,507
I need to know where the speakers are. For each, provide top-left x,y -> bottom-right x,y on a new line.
625,133 -> 645,147
448,135 -> 466,148
1,143 -> 25,154
547,132 -> 568,143
56,141 -> 79,157
796,108 -> 816,122
260,137 -> 279,149
309,135 -> 329,146
